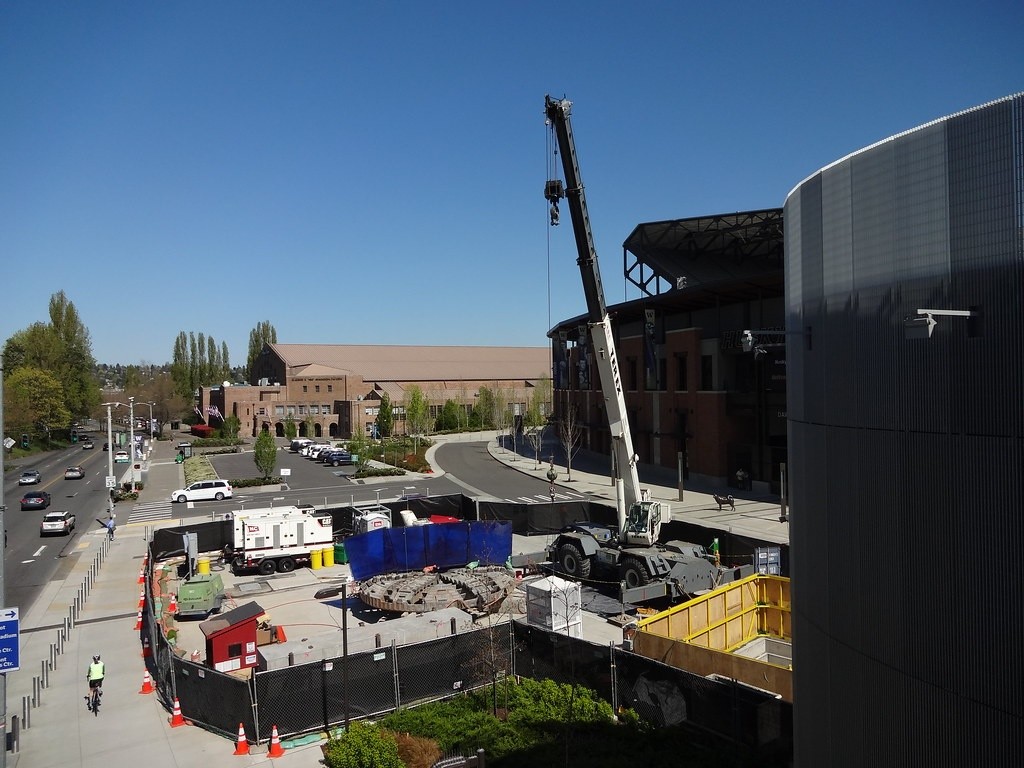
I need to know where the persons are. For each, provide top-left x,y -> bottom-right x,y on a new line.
736,467 -> 750,493
107,516 -> 114,541
87,654 -> 107,707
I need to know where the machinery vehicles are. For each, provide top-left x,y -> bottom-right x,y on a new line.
540,94 -> 681,605
227,506 -> 335,576
176,569 -> 224,619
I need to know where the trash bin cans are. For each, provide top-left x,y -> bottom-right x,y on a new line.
197,558 -> 210,573
334,544 -> 348,565
323,547 -> 334,566
309,550 -> 322,570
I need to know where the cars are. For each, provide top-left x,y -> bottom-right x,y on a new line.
80,434 -> 93,449
40,511 -> 76,534
103,442 -> 113,451
290,437 -> 356,466
20,470 -> 40,485
65,466 -> 85,480
20,491 -> 52,510
115,451 -> 130,463
171,477 -> 232,504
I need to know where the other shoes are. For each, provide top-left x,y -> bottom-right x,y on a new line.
98,700 -> 102,705
88,703 -> 92,707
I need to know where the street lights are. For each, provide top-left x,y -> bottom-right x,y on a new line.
102,397 -> 154,517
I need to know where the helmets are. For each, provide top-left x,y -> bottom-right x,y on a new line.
92,655 -> 100,660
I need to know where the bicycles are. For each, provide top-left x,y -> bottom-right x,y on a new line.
90,683 -> 100,715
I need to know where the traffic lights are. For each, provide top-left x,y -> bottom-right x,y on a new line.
71,428 -> 78,443
21,433 -> 29,449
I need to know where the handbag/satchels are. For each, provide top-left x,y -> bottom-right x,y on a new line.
111,526 -> 116,531
